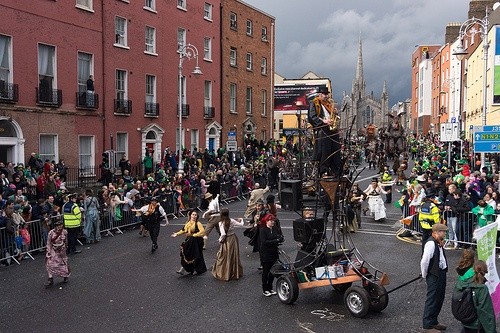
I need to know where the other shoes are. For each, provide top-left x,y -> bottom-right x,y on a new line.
64,277 -> 69,282
46,280 -> 53,287
151,243 -> 158,251
422,328 -> 440,333
434,323 -> 447,330
262,291 -> 271,297
176,268 -> 185,275
270,290 -> 277,294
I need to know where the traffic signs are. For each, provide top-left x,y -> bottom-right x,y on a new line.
227,141 -> 238,152
473,125 -> 500,153
440,122 -> 460,142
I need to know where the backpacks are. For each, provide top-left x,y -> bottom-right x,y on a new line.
451,278 -> 484,324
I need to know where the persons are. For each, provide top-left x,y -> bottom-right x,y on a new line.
455,250 -> 481,333
0,138 -> 84,258
84,190 -> 102,245
461,260 -> 498,333
171,209 -> 207,277
244,200 -> 269,268
39,75 -> 50,101
300,207 -> 314,223
211,208 -> 244,282
258,214 -> 281,296
86,74 -> 94,108
97,134 -> 315,238
420,223 -> 449,330
307,85 -> 342,177
345,133 -> 500,251
45,219 -> 72,288
128,197 -> 170,252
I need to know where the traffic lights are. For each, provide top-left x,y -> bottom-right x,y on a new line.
102,152 -> 110,169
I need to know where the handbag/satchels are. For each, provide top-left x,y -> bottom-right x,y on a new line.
218,235 -> 227,243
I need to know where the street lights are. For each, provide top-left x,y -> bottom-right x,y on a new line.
178,43 -> 203,178
295,101 -> 303,180
452,5 -> 487,173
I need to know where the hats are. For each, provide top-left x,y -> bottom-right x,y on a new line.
316,86 -> 330,94
204,192 -> 212,200
255,183 -> 259,186
68,194 -> 77,198
118,188 -> 124,192
54,221 -> 63,225
432,224 -> 447,231
255,198 -> 264,204
